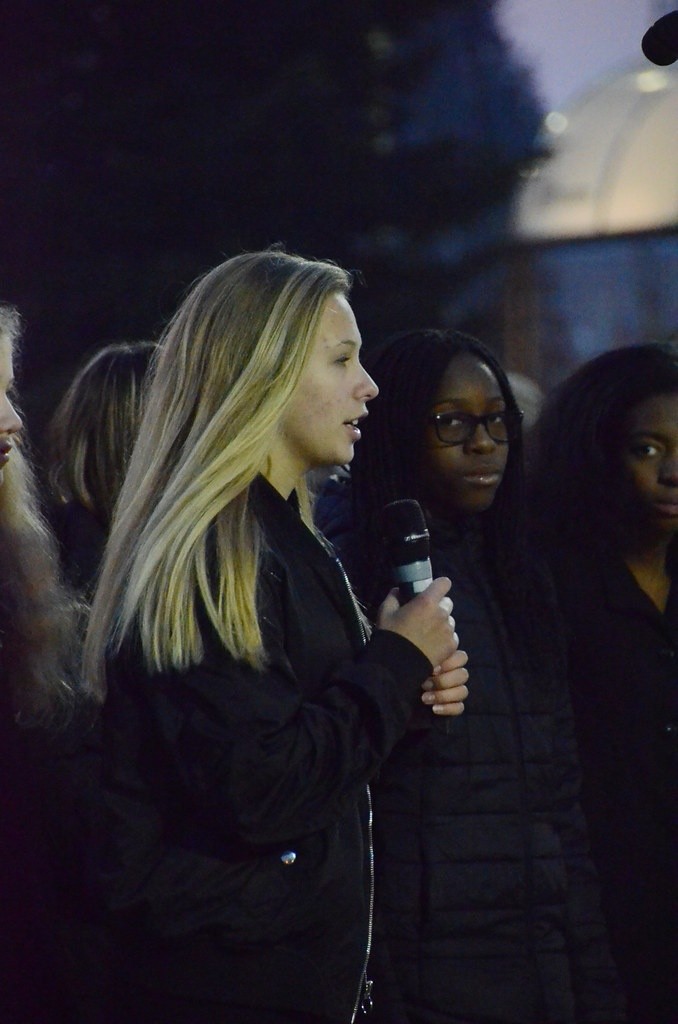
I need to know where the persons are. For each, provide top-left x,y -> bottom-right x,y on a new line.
1,299 -> 164,1024
356,327 -> 628,1024
527,342 -> 678,1023
53,248 -> 473,1024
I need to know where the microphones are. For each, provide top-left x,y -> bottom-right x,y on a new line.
383,497 -> 433,604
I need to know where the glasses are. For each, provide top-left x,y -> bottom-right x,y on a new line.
426,410 -> 525,444
